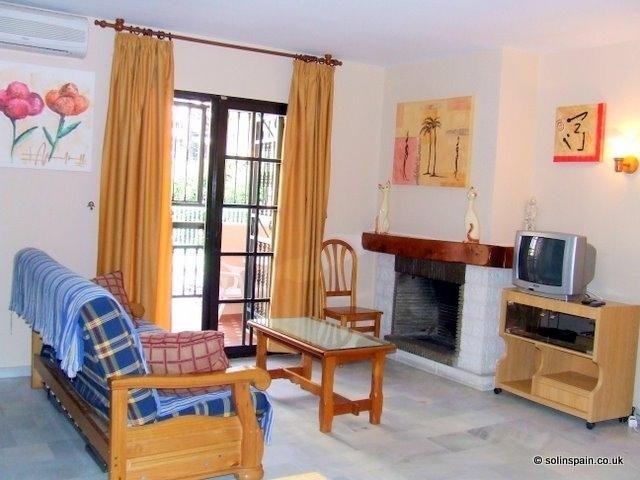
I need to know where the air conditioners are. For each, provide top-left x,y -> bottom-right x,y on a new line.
0,2 -> 87,58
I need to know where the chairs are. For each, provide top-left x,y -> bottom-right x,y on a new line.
199,250 -> 251,326
318,239 -> 383,339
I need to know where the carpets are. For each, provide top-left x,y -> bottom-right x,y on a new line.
269,471 -> 326,480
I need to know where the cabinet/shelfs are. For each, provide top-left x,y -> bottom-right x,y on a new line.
495,287 -> 640,420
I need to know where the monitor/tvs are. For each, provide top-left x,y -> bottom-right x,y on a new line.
511,228 -> 599,301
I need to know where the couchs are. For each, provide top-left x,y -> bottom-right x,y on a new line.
11,247 -> 271,480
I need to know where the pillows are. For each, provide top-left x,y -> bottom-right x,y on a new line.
86,270 -> 136,323
137,330 -> 233,396
